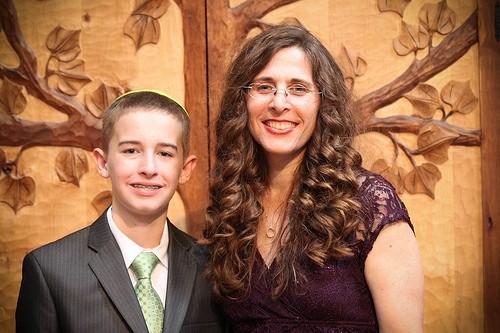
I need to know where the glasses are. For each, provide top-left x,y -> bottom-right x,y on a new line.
243,83 -> 323,100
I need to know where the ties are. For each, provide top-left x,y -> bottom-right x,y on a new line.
129,252 -> 164,333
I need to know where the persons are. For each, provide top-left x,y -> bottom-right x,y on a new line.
203,23 -> 424,333
15,88 -> 219,333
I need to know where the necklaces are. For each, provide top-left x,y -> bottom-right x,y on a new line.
261,202 -> 281,238
